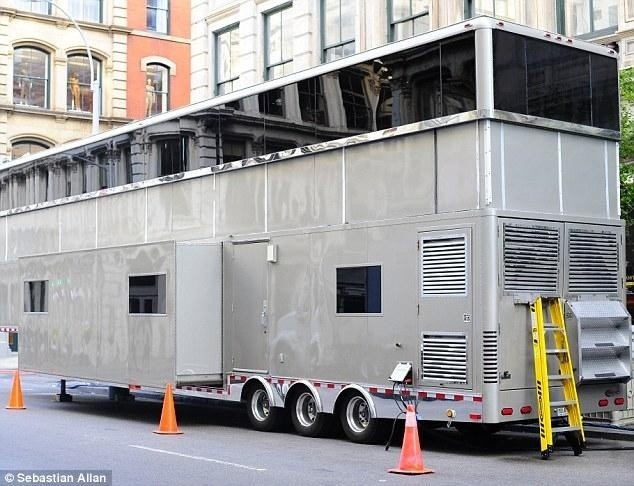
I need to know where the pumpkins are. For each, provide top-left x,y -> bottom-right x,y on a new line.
528,295 -> 588,459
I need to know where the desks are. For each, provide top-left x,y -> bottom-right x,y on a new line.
21,0 -> 99,136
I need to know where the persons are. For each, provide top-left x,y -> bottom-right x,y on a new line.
16,61 -> 33,106
68,71 -> 83,111
145,77 -> 155,118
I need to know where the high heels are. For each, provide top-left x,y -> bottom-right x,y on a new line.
386,404 -> 434,475
152,384 -> 184,435
4,370 -> 26,409
